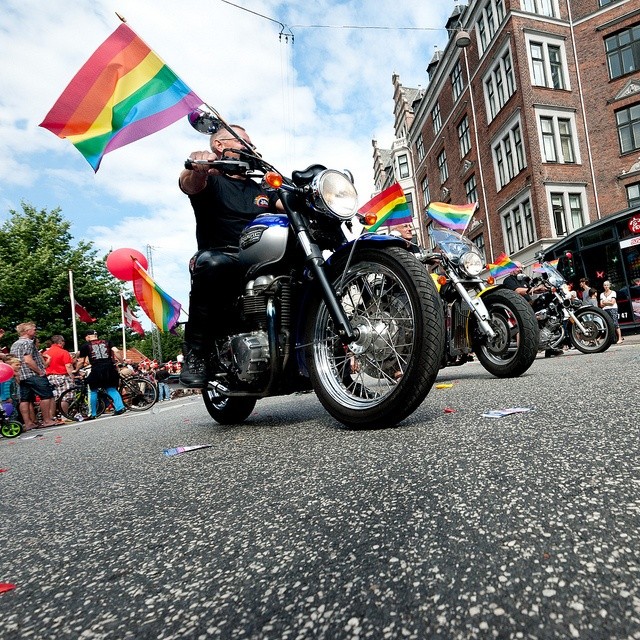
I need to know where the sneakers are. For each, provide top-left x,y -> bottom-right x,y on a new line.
114,408 -> 125,415
83,416 -> 94,421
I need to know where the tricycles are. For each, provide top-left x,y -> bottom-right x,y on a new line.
0,393 -> 23,438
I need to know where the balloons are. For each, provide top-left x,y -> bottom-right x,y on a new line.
106,248 -> 149,282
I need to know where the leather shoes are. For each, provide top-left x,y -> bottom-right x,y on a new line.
179,349 -> 209,387
344,382 -> 380,399
545,349 -> 563,358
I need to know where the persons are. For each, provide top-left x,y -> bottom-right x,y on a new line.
39,340 -> 52,352
579,278 -> 591,301
118,362 -> 158,384
178,126 -> 287,388
583,289 -> 598,308
72,330 -> 127,421
10,322 -> 64,431
386,222 -> 439,313
33,338 -> 41,351
155,365 -> 170,402
600,281 -> 623,344
0,328 -> 22,421
503,260 -> 564,358
43,335 -> 75,422
562,283 -> 577,350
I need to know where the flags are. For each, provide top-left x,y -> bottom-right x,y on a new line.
38,23 -> 205,173
74,297 -> 96,323
532,260 -> 558,273
121,295 -> 144,335
355,182 -> 412,234
427,201 -> 475,230
485,252 -> 519,279
133,257 -> 181,337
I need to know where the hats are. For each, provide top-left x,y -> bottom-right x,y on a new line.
159,364 -> 165,368
86,330 -> 98,336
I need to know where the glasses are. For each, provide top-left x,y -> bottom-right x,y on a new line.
220,136 -> 256,152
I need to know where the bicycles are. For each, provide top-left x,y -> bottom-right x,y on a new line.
57,362 -> 157,422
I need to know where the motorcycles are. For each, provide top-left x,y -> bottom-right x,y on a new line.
499,259 -> 614,359
168,107 -> 446,430
348,221 -> 539,379
